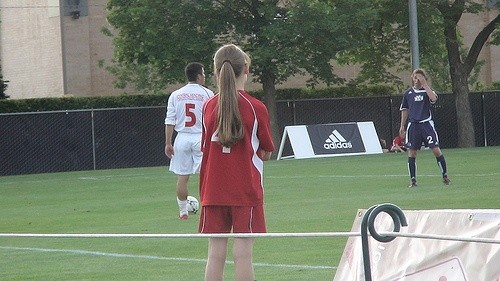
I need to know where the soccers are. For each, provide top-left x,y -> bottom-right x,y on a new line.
187,195 -> 199,215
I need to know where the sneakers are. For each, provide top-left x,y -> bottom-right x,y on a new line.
179,210 -> 189,220
409,183 -> 417,188
443,178 -> 451,185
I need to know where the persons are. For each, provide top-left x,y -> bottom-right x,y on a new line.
165,62 -> 215,219
399,69 -> 452,188
389,132 -> 407,153
198,44 -> 275,281
417,141 -> 429,151
